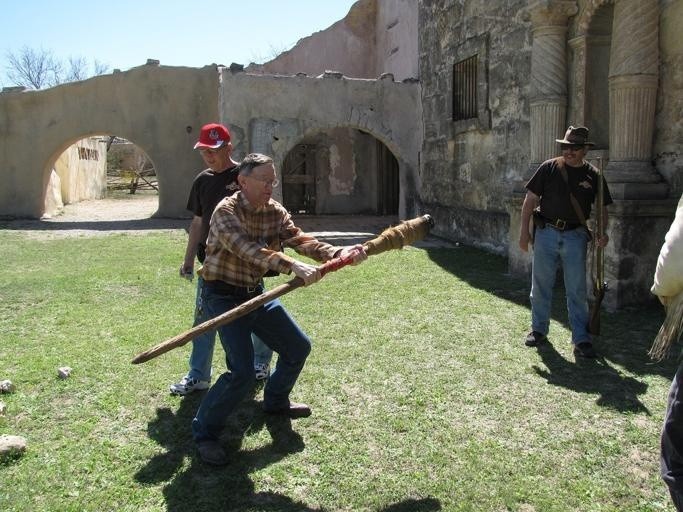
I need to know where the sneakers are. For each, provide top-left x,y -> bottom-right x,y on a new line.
526,327 -> 549,346
170,374 -> 209,395
255,362 -> 269,380
575,342 -> 594,357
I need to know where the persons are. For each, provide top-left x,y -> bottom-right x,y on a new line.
646,190 -> 683,512
190,152 -> 368,460
518,125 -> 614,358
169,122 -> 274,395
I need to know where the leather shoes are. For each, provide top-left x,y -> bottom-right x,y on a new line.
198,441 -> 228,465
262,400 -> 311,413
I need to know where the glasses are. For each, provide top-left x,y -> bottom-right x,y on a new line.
560,145 -> 583,151
199,144 -> 227,156
248,175 -> 279,188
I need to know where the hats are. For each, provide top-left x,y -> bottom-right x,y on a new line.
194,123 -> 230,150
555,126 -> 595,145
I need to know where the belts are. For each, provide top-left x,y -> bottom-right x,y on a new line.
239,286 -> 255,294
545,219 -> 579,230
